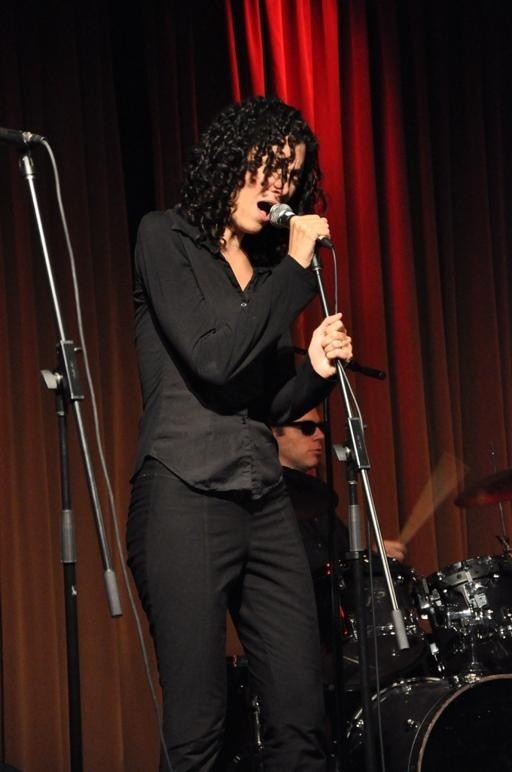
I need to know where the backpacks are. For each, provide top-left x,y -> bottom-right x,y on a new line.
416,553 -> 512,681
315,553 -> 430,693
335,673 -> 512,772
159,654 -> 267,772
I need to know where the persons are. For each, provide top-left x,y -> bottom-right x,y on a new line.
123,96 -> 356,770
273,397 -> 413,658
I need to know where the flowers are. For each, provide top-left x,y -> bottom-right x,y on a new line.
0,127 -> 45,145
270,202 -> 333,250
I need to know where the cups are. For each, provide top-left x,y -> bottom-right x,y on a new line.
283,421 -> 330,435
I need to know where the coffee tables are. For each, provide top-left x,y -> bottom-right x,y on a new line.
454,469 -> 512,507
